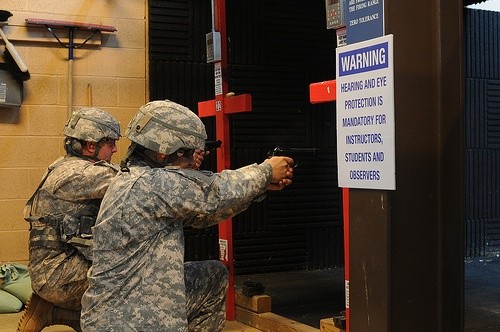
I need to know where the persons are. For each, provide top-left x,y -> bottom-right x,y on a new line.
16,107 -> 210,332
81,99 -> 294,332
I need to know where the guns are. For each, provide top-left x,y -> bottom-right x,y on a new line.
198,140 -> 222,155
266,144 -> 322,185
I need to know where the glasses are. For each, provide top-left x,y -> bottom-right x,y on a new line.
179,149 -> 196,160
102,136 -> 116,146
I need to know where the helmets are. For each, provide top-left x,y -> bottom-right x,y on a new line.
64,108 -> 122,142
126,100 -> 207,156
